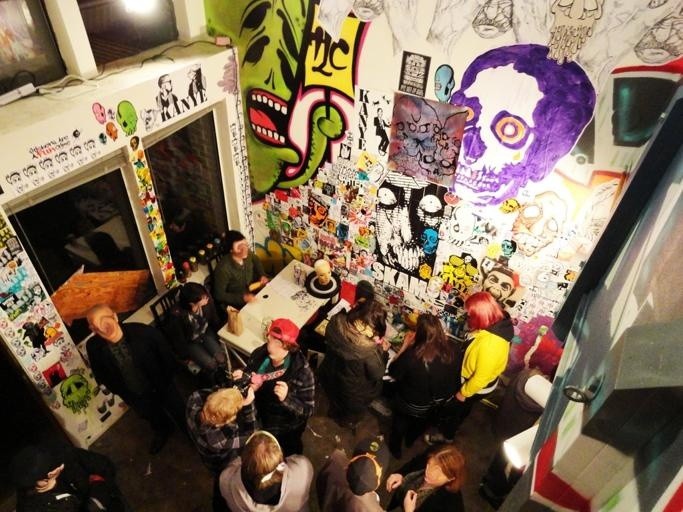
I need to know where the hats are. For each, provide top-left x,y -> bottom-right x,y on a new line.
269,318 -> 299,347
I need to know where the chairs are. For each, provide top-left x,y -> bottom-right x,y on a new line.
149,248 -> 223,323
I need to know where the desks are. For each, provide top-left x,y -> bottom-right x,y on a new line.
216,258 -> 330,371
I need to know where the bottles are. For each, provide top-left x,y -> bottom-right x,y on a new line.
261,318 -> 272,342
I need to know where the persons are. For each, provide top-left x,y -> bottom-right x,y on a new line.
86,231 -> 514,512
13,445 -> 136,512
